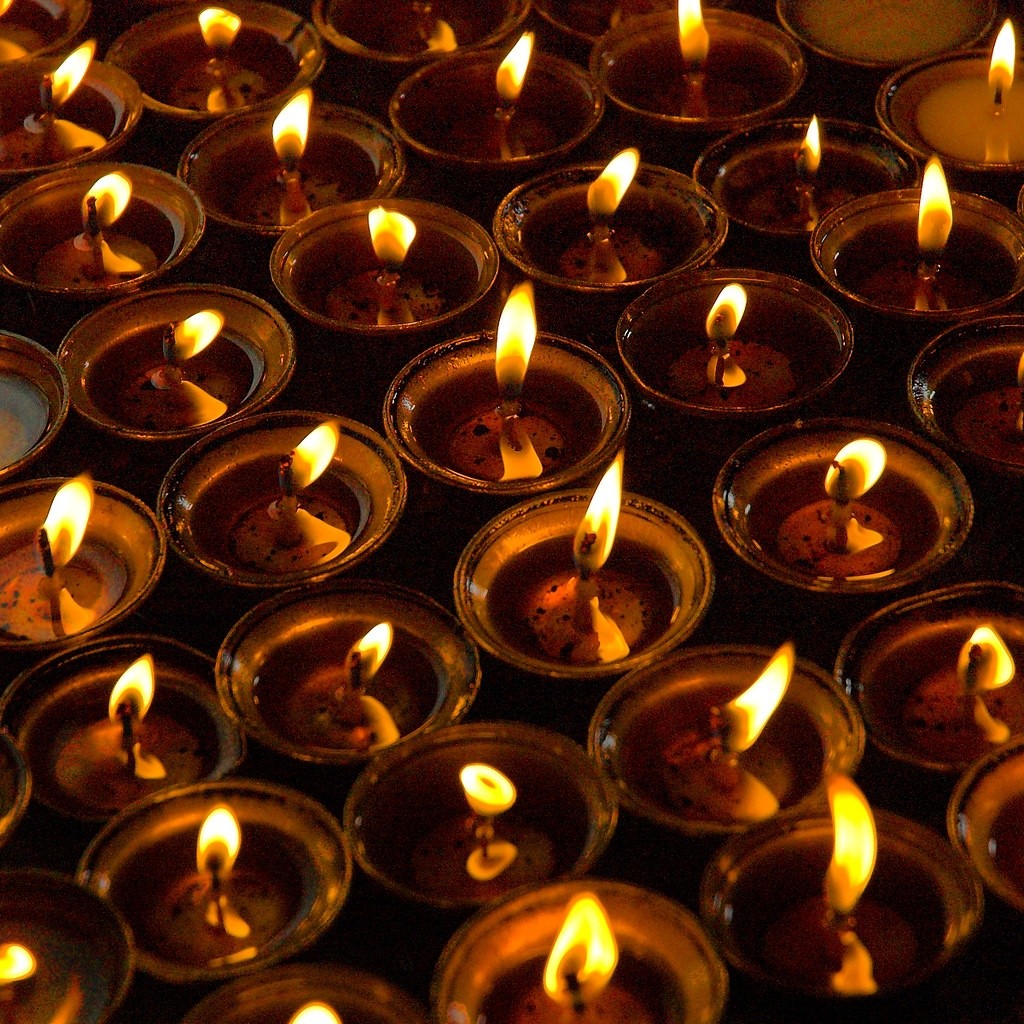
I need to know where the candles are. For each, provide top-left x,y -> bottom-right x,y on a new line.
0,0 -> 1024,1023
